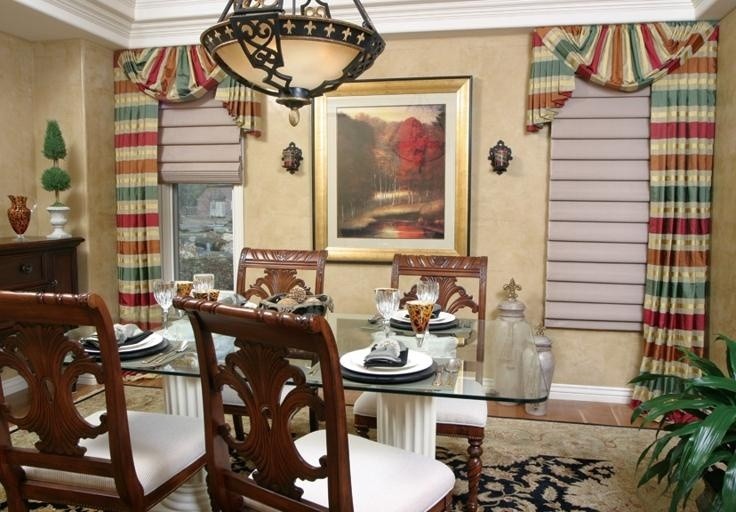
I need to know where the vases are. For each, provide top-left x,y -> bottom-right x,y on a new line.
45,207 -> 73,239
6,194 -> 32,243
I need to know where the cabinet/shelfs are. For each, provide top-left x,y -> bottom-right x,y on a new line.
0,235 -> 86,392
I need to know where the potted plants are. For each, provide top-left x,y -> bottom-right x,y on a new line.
624,330 -> 736,512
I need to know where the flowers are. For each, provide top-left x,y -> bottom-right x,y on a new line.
40,118 -> 72,206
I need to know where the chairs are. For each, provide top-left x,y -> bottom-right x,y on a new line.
171,295 -> 456,512
218,245 -> 330,440
0,291 -> 231,512
351,253 -> 488,512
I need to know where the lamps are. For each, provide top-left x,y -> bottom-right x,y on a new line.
198,0 -> 387,129
279,142 -> 304,176
488,138 -> 513,175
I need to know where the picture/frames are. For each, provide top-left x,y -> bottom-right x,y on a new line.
312,75 -> 472,266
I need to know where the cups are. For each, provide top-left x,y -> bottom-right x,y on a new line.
193,273 -> 215,289
175,281 -> 194,298
404,298 -> 436,357
415,281 -> 441,346
153,279 -> 178,335
192,289 -> 220,303
374,288 -> 401,351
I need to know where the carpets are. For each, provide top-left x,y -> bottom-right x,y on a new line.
0,381 -> 736,512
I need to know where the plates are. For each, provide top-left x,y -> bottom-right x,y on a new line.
77,332 -> 163,352
70,338 -> 170,362
390,318 -> 460,331
339,346 -> 435,377
340,360 -> 439,384
393,309 -> 456,325
